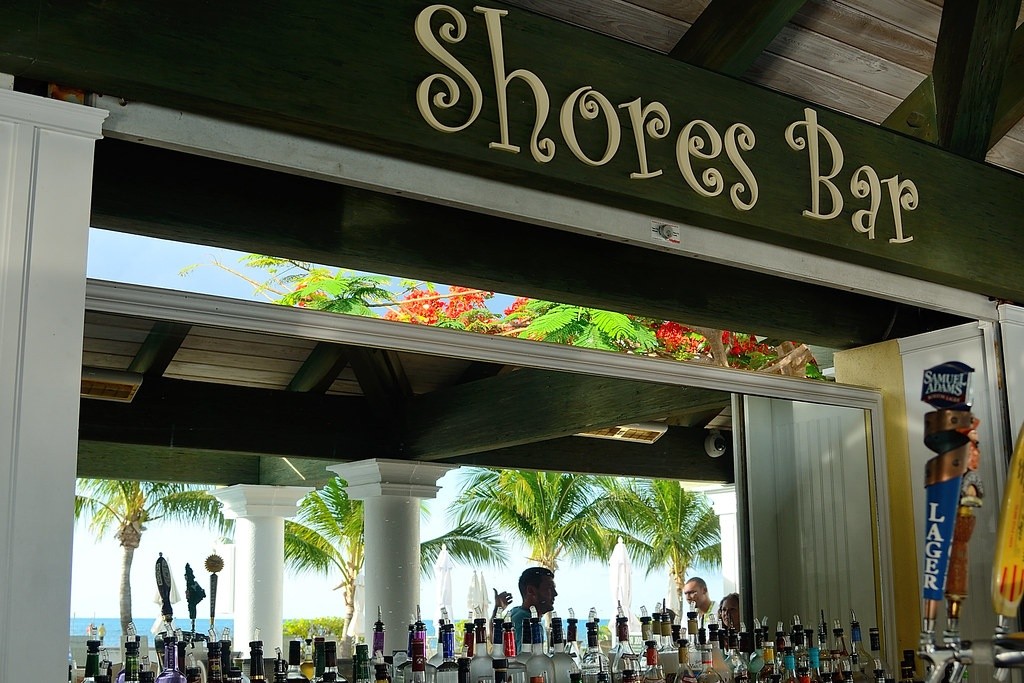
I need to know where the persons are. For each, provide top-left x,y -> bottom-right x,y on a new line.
485,567 -> 559,658
716,593 -> 739,650
682,576 -> 720,639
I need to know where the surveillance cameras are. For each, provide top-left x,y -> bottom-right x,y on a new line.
704,429 -> 727,458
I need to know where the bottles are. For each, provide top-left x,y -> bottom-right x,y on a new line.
69,599 -> 918,682
